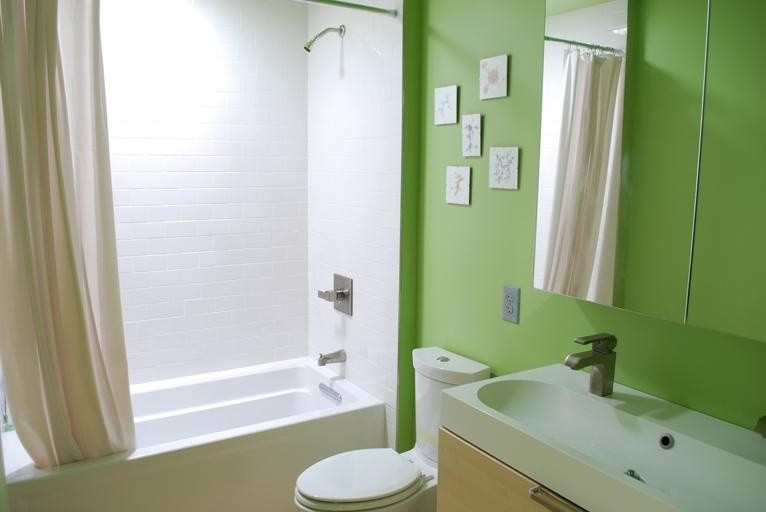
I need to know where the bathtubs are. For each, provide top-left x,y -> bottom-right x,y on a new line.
0,356 -> 390,512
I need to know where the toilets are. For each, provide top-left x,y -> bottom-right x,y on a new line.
293,346 -> 491,512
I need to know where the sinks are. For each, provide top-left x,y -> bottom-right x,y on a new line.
441,362 -> 766,512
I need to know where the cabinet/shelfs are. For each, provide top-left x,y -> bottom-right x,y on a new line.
434,427 -> 587,512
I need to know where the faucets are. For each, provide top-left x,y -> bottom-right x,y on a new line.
563,333 -> 618,397
318,349 -> 346,366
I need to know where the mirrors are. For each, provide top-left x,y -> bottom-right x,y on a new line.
538,0 -> 632,303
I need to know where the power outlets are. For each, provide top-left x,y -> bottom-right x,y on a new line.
502,285 -> 520,324
